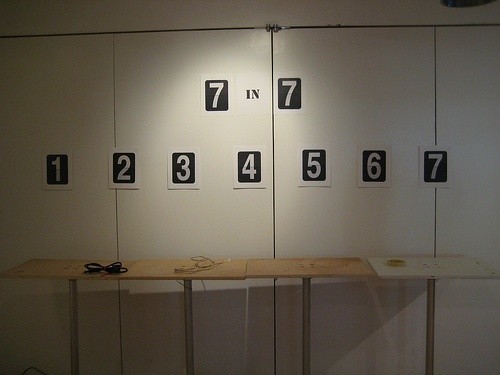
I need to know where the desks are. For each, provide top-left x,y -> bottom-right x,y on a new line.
0,256 -> 500,375
